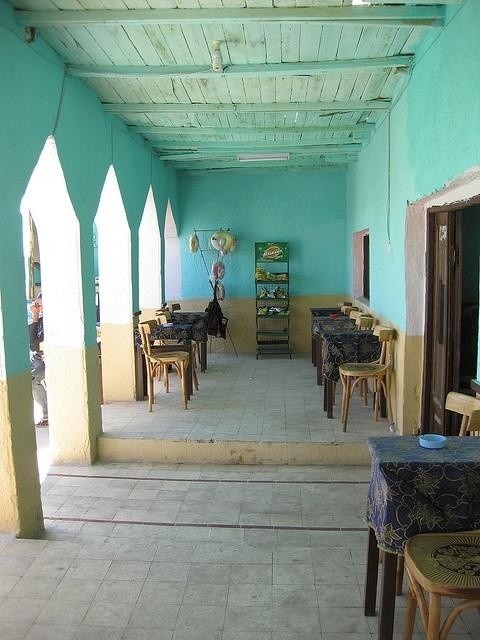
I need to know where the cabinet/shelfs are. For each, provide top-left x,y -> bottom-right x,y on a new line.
254,241 -> 291,360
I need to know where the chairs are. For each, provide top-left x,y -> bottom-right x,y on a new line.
333,316 -> 376,406
445,391 -> 480,434
137,304 -> 208,412
402,530 -> 480,640
310,302 -> 371,385
339,325 -> 395,433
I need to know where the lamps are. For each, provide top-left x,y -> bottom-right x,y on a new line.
239,153 -> 289,162
213,40 -> 223,72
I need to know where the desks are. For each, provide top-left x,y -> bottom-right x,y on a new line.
365,432 -> 480,639
322,330 -> 382,418
